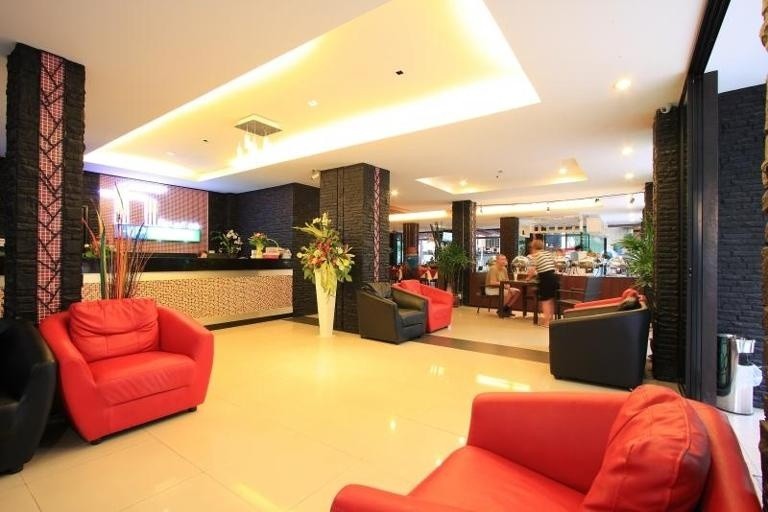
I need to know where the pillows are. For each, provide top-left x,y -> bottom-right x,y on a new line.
362,279 -> 422,301
621,289 -> 639,298
616,297 -> 640,312
70,296 -> 159,363
579,382 -> 710,512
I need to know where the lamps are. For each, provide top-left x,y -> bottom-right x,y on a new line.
235,114 -> 283,159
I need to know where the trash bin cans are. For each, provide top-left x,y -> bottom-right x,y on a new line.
716,333 -> 756,416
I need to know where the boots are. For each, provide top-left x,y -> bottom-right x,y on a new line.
497,305 -> 515,318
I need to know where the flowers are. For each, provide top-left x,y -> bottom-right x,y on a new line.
290,213 -> 355,297
248,232 -> 268,250
210,230 -> 241,253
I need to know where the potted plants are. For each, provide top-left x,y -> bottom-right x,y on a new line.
436,241 -> 471,307
611,208 -> 654,359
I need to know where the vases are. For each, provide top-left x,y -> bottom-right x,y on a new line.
314,269 -> 338,336
256,246 -> 262,258
226,250 -> 238,258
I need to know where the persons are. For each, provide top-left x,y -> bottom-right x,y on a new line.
555,245 -> 603,275
484,253 -> 522,319
524,239 -> 556,328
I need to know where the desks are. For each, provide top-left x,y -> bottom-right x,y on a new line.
389,250 -> 624,324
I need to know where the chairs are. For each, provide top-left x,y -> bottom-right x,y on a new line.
39,312 -> 214,446
575,294 -> 647,302
0,317 -> 57,477
356,283 -> 454,345
549,301 -> 650,391
331,390 -> 764,512
